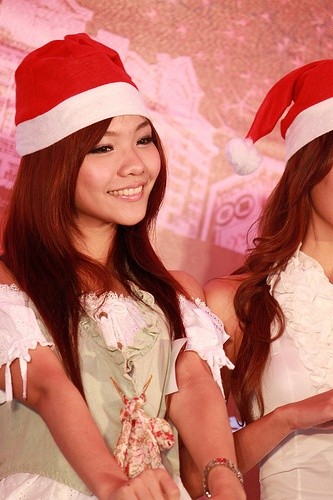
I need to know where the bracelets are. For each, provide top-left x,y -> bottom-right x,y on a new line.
203,455 -> 245,496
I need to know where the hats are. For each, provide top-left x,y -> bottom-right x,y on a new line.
226,59 -> 333,176
14,32 -> 152,158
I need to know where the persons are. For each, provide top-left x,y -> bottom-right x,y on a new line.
1,33 -> 247,500
179,59 -> 333,500
